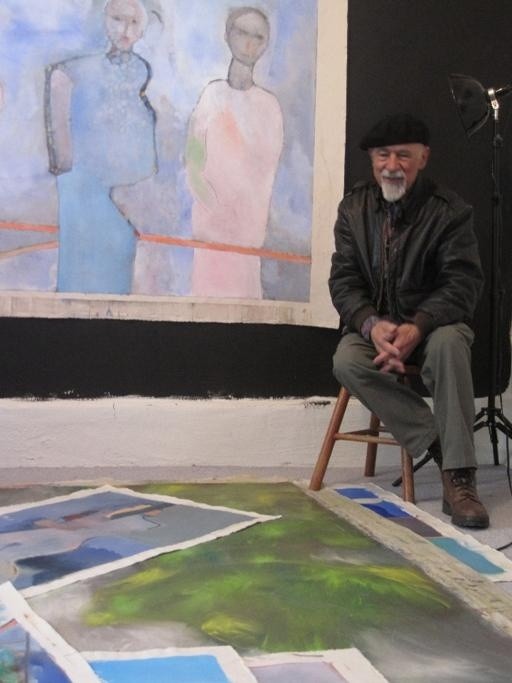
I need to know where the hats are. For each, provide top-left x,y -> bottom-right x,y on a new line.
360,113 -> 429,152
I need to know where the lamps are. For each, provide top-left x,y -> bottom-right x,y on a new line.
394,69 -> 512,490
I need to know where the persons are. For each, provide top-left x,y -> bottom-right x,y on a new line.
330,113 -> 490,530
45,0 -> 160,296
183,7 -> 283,299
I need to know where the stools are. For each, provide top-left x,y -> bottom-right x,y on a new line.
310,376 -> 425,505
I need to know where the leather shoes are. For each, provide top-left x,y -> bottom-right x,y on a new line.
442,468 -> 489,528
